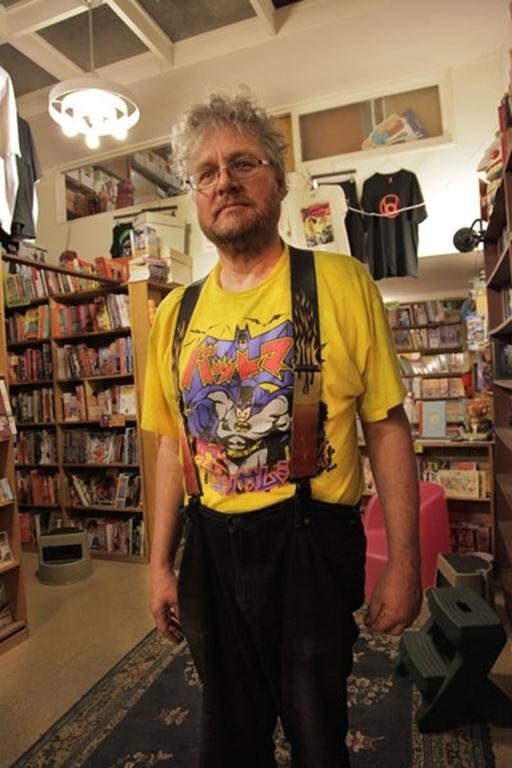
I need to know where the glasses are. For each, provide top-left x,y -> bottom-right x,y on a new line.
185,157 -> 270,192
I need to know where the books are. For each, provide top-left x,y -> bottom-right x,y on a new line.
0,246 -> 193,566
361,292 -> 494,554
477,80 -> 512,379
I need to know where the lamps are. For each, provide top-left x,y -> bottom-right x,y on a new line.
47,0 -> 142,147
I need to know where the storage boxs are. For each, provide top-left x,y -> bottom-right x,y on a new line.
129,208 -> 196,290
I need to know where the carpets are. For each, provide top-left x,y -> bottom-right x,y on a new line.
10,588 -> 494,768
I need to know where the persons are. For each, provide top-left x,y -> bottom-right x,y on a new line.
141,87 -> 424,767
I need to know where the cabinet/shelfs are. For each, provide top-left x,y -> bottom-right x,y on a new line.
477,126 -> 509,768
6,250 -> 488,570
0,391 -> 30,657
